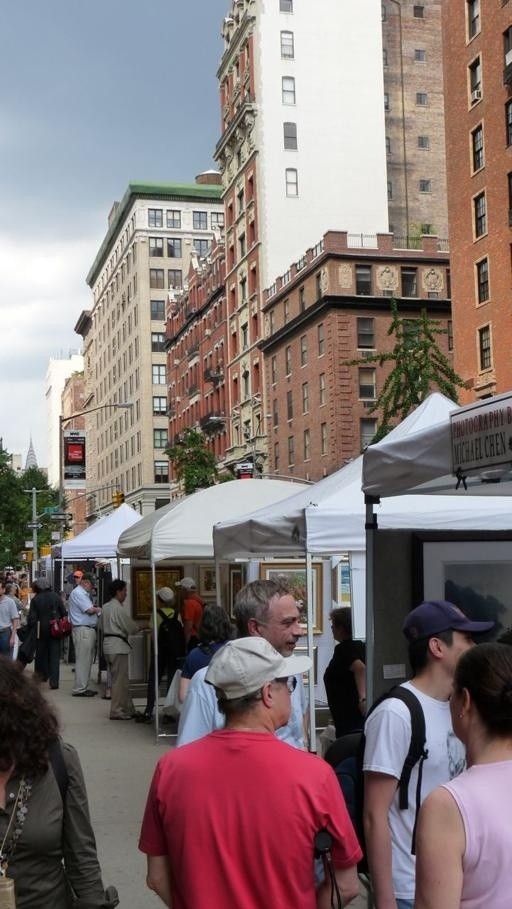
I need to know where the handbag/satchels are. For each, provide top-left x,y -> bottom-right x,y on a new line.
49,616 -> 71,638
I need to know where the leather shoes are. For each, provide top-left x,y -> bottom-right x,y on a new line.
111,711 -> 153,723
74,691 -> 98,697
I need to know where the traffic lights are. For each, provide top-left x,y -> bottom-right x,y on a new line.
117,490 -> 125,507
20,550 -> 33,563
112,492 -> 119,509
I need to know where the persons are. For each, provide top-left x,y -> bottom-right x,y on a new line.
135,581 -> 511,908
0,658 -> 121,909
1,570 -> 141,721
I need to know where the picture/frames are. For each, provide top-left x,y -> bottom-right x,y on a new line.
328,555 -> 353,618
130,566 -> 186,621
197,563 -> 217,599
226,564 -> 245,623
258,559 -> 324,636
407,527 -> 512,645
292,646 -> 319,687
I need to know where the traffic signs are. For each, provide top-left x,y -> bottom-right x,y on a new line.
49,513 -> 73,520
26,522 -> 44,530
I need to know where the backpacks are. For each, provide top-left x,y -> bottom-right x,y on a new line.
321,685 -> 426,848
156,608 -> 185,662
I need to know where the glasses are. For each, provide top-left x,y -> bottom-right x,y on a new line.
285,676 -> 298,692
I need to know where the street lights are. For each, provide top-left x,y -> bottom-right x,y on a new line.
56,402 -> 134,543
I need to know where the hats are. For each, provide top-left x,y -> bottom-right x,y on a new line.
401,600 -> 496,640
175,577 -> 198,592
203,637 -> 315,700
74,571 -> 97,585
156,587 -> 175,602
36,576 -> 51,589
